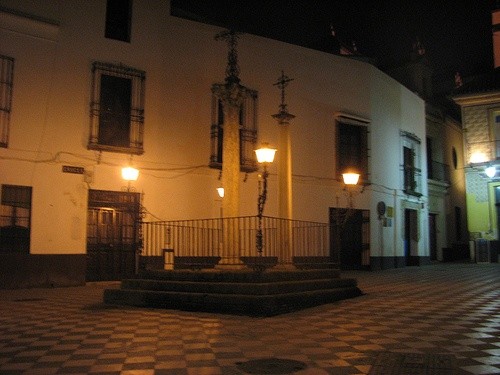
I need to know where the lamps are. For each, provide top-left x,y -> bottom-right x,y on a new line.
253,142 -> 279,216
121,164 -> 139,207
338,165 -> 362,220
